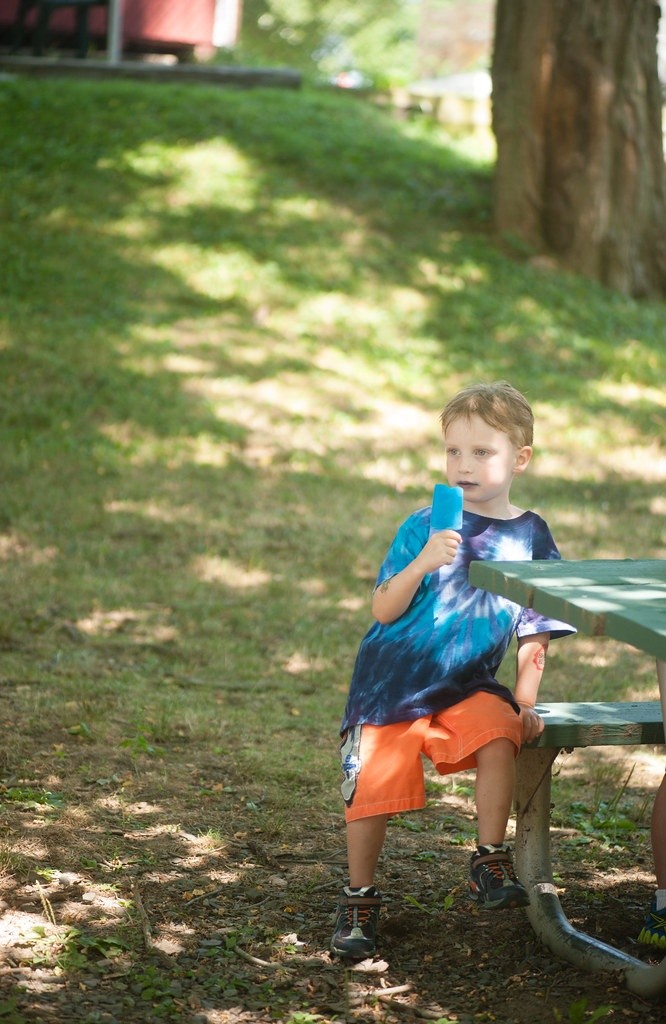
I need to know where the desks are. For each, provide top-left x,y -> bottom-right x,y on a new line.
471,558 -> 666,656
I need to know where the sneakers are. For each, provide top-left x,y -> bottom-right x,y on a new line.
331,886 -> 382,959
467,843 -> 531,910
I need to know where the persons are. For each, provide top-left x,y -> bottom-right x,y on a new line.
332,383 -> 578,962
639,773 -> 666,955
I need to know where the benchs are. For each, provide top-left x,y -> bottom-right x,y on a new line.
507,698 -> 665,997
